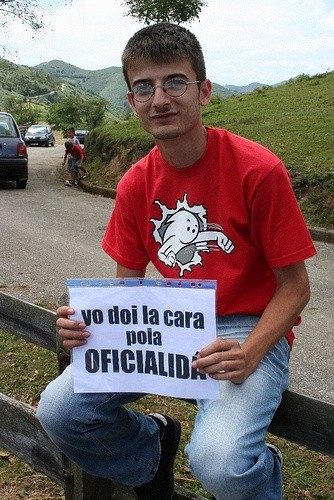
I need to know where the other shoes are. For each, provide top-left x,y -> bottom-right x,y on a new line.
133,412 -> 181,500
83,172 -> 89,178
265,442 -> 283,468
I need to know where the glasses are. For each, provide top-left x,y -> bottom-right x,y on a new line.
126,79 -> 202,102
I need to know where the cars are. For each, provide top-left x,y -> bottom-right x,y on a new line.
24,124 -> 55,147
0,111 -> 28,189
74,129 -> 89,144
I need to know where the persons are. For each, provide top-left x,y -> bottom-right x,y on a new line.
37,21 -> 317,500
66,128 -> 81,183
62,141 -> 89,184
